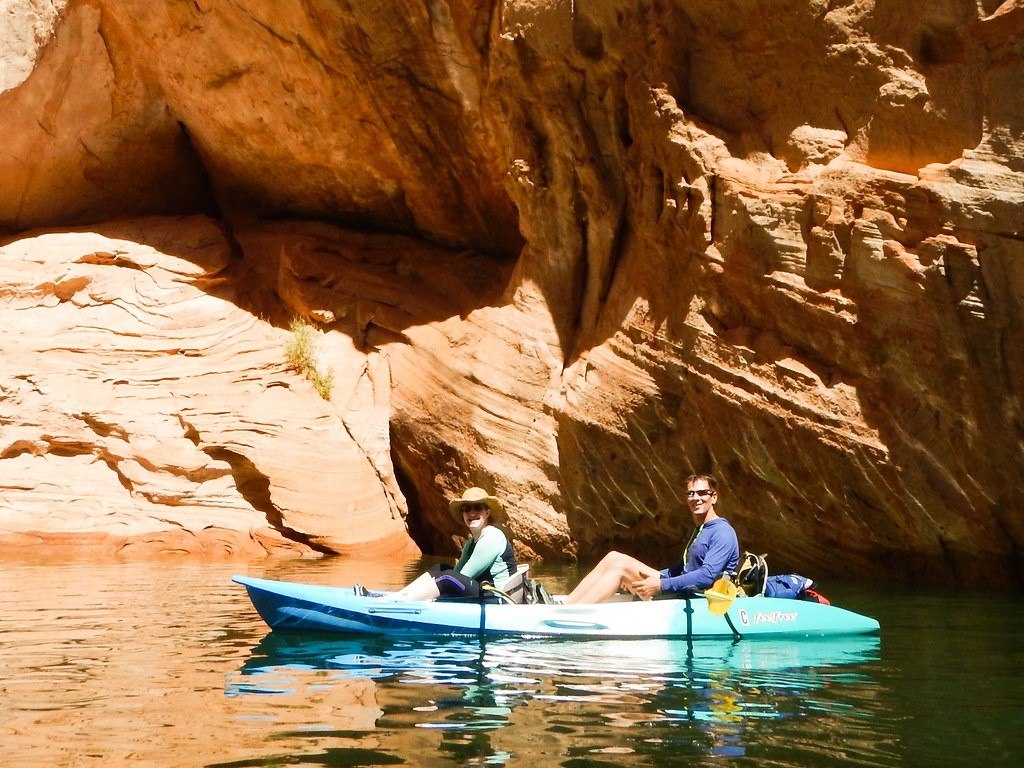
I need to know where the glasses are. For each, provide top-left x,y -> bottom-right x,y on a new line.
686,490 -> 712,496
461,503 -> 488,513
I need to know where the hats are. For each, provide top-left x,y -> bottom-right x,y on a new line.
449,487 -> 503,525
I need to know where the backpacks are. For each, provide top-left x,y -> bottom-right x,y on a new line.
735,551 -> 769,597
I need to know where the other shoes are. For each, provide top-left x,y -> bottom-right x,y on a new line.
524,580 -> 538,604
536,584 -> 553,604
354,581 -> 381,597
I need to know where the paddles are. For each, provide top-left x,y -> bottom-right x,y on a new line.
454,558 -> 518,605
689,579 -> 737,615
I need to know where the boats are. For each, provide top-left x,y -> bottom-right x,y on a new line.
230,573 -> 884,645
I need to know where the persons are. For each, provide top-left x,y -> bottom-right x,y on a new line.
355,487 -> 516,604
524,474 -> 738,605
